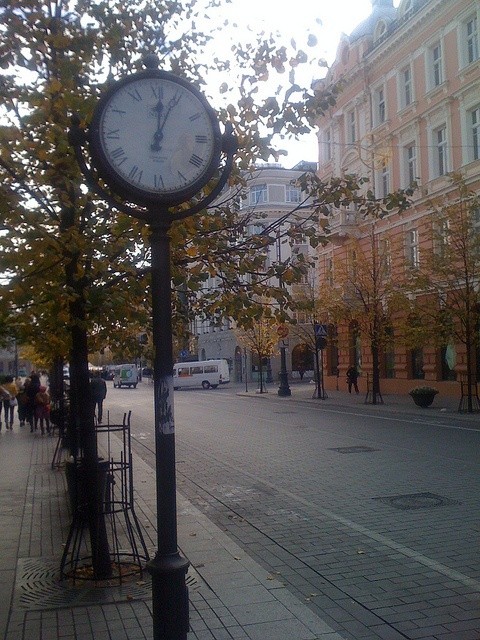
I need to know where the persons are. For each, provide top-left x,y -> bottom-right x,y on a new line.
346,364 -> 360,393
1,376 -> 19,430
34,385 -> 50,436
90,370 -> 106,423
22,375 -> 41,433
16,377 -> 25,428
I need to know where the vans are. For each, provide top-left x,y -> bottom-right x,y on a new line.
173,359 -> 230,391
113,363 -> 139,389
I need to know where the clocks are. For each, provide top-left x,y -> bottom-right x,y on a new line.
88,69 -> 235,209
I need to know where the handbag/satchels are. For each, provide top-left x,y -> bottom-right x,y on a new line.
1,385 -> 17,407
